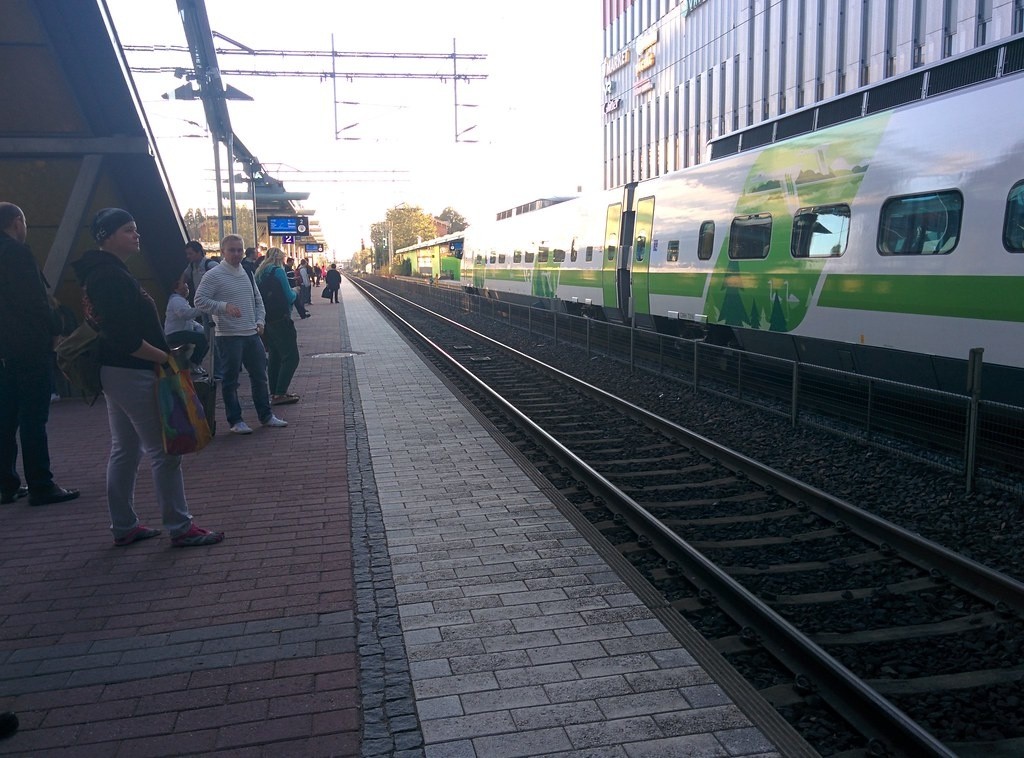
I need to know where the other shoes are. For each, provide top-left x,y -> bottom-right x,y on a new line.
187,359 -> 206,373
0,712 -> 19,738
202,374 -> 222,380
301,313 -> 311,319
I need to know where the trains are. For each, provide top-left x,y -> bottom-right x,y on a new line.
459,72 -> 1024,441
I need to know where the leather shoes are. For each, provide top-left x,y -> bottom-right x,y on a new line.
30,484 -> 80,506
0,486 -> 28,502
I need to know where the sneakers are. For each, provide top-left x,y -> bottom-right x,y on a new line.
271,393 -> 299,405
227,421 -> 253,434
172,521 -> 225,546
260,414 -> 288,427
113,524 -> 161,546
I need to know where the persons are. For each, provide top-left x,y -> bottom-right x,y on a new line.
162,278 -> 209,374
0,201 -> 80,505
177,235 -> 321,434
74,206 -> 225,546
322,263 -> 341,303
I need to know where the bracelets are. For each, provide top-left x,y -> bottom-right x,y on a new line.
161,360 -> 170,370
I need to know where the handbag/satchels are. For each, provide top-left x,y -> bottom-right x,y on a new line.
294,267 -> 303,286
322,287 -> 330,298
50,305 -> 76,338
154,355 -> 214,456
258,267 -> 291,322
53,314 -> 107,406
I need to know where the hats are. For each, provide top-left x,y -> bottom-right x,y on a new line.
301,259 -> 308,264
90,208 -> 135,243
